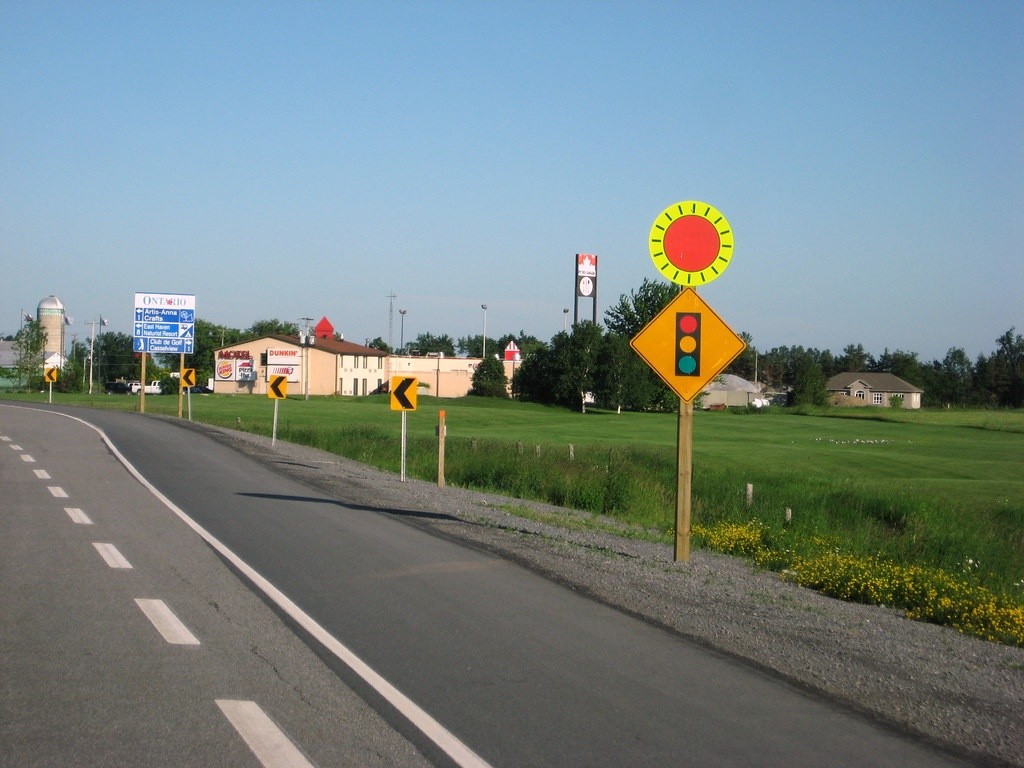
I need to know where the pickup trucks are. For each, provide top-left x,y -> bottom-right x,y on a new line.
132,381 -> 162,396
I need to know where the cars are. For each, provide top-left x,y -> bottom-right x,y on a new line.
183,386 -> 213,394
104,382 -> 131,395
